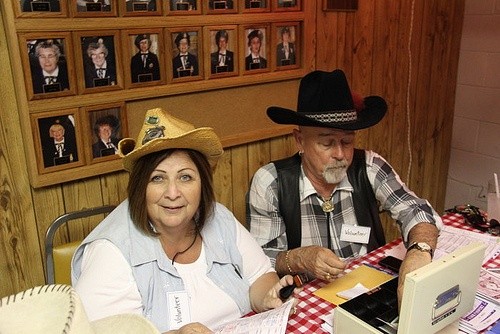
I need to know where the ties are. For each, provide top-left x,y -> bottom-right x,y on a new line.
180,55 -> 188,69
57,144 -> 63,157
106,141 -> 116,151
44,76 -> 57,84
96,68 -> 106,78
140,53 -> 148,68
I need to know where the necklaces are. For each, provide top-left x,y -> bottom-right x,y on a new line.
172,217 -> 197,266
314,192 -> 337,212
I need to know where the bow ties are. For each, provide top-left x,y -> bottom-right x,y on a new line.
218,53 -> 225,64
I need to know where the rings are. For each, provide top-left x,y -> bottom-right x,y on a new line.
325,273 -> 330,280
292,307 -> 297,314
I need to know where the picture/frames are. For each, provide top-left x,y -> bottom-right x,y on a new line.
11,0 -> 317,175
322,0 -> 359,12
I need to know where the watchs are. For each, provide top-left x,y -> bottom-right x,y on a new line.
406,241 -> 434,259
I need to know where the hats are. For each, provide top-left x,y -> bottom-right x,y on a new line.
266,69 -> 388,130
282,27 -> 290,33
216,30 -> 229,42
94,115 -> 119,135
117,107 -> 225,173
247,29 -> 264,46
47,118 -> 67,130
134,34 -> 150,49
174,33 -> 190,47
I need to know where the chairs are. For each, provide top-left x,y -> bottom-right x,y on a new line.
44,204 -> 117,285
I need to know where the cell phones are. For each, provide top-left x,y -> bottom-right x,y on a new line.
379,256 -> 402,272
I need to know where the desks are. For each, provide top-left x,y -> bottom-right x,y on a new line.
242,209 -> 500,334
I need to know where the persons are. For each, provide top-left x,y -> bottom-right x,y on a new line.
131,33 -> 160,83
71,106 -> 299,334
245,29 -> 267,71
85,43 -> 117,89
32,39 -> 70,93
92,115 -> 121,158
173,33 -> 198,78
43,124 -> 78,168
276,26 -> 296,66
248,70 -> 442,318
211,29 -> 234,74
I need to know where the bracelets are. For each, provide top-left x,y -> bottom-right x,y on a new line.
286,250 -> 297,276
